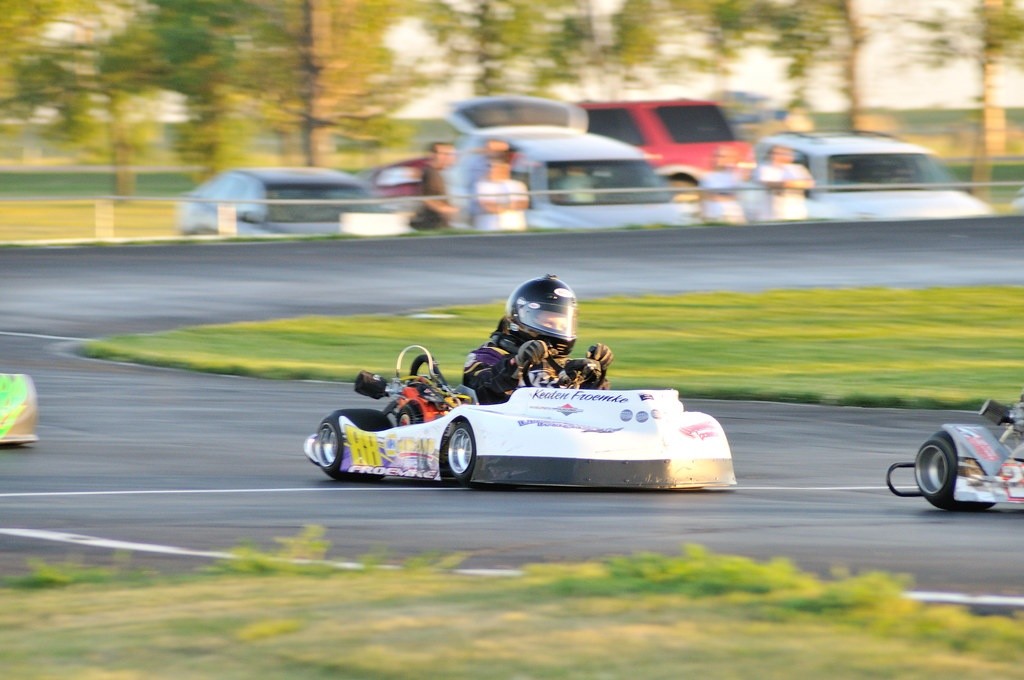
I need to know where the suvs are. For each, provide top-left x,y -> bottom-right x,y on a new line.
579,95 -> 757,205
439,95 -> 696,232
754,126 -> 990,218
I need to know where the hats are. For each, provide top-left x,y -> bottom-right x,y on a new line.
478,140 -> 520,158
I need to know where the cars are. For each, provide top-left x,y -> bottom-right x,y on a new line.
178,167 -> 393,235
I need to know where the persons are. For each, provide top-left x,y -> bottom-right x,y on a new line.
699,145 -> 816,220
470,157 -> 531,232
411,140 -> 459,231
462,274 -> 613,406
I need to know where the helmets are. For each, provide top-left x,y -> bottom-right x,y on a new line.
505,274 -> 578,355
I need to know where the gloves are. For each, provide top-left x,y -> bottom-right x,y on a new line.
585,343 -> 614,370
514,339 -> 549,367
